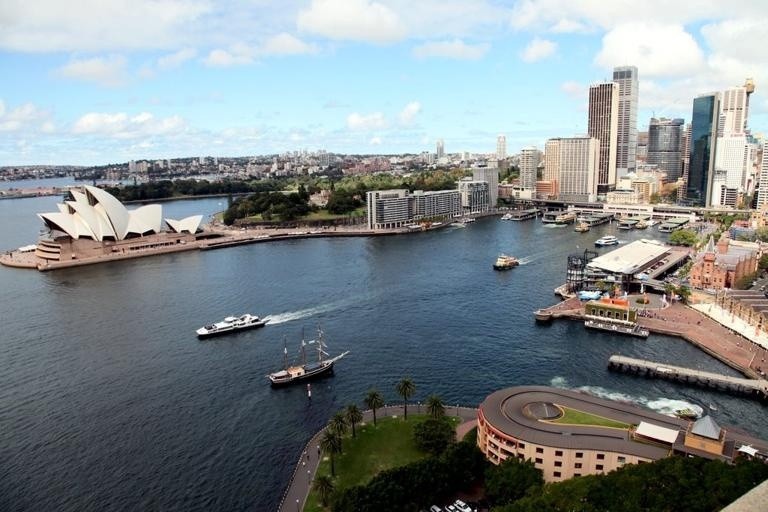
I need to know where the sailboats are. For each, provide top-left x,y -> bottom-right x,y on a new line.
265,322 -> 351,383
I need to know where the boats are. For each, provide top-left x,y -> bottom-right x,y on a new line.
490,253 -> 519,271
594,234 -> 618,247
501,212 -> 691,233
672,408 -> 698,417
196,314 -> 272,337
458,217 -> 476,223
